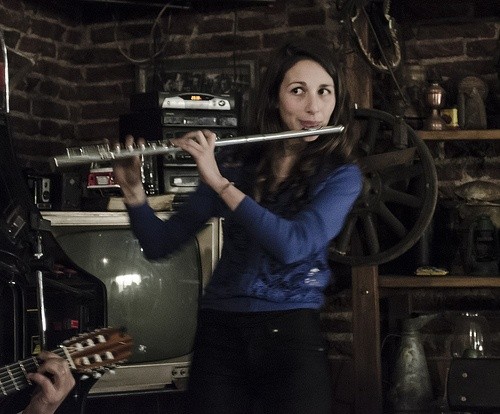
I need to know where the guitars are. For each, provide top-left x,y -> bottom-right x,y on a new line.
0,325 -> 134,398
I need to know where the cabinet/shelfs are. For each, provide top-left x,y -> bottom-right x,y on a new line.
347,3 -> 500,414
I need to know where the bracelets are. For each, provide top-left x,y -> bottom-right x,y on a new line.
218,181 -> 236,197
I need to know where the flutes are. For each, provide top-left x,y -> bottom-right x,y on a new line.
54,126 -> 344,167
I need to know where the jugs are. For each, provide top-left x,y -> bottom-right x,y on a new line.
383,310 -> 440,412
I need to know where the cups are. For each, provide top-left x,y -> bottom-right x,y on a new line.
451,313 -> 492,360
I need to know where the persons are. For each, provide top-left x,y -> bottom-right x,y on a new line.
102,41 -> 366,414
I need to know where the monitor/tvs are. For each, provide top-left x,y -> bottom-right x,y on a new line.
40,211 -> 224,394
